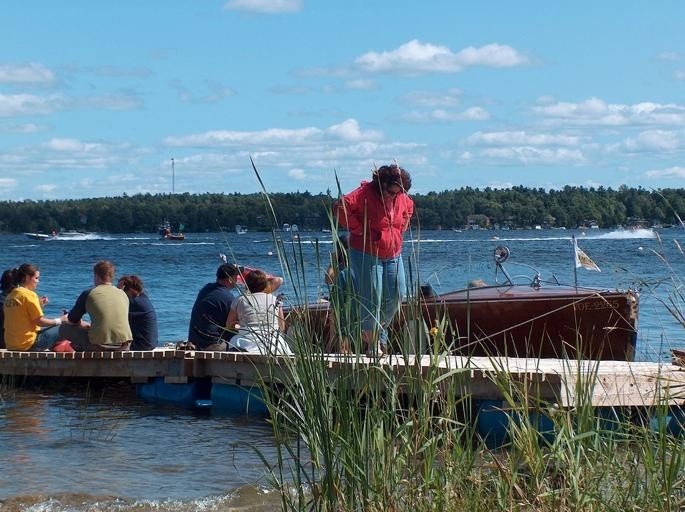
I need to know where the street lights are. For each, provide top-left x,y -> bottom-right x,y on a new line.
171,158 -> 174,194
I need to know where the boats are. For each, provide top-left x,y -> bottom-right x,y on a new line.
58,232 -> 85,237
24,232 -> 48,240
282,234 -> 640,361
164,233 -> 183,240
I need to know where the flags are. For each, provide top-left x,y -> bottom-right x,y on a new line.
574,237 -> 604,273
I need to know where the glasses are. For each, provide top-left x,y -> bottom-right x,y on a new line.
386,186 -> 402,195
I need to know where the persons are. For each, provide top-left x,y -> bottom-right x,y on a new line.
186,263 -> 293,356
0,261 -> 159,352
329,163 -> 418,359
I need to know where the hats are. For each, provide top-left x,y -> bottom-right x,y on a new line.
215,262 -> 245,280
